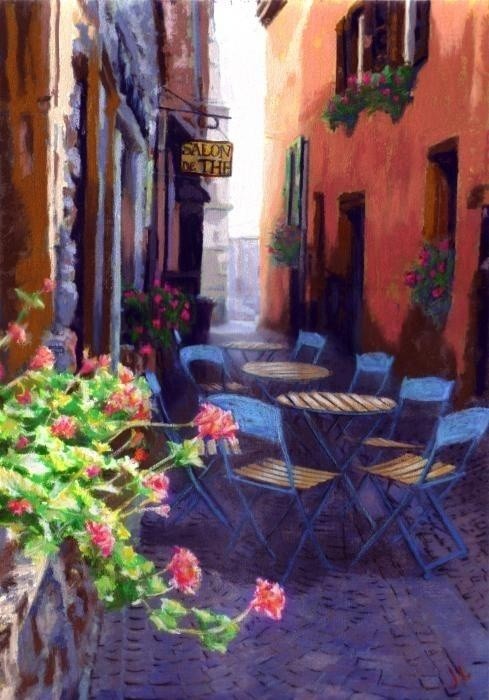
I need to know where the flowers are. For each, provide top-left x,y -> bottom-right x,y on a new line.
321,64 -> 412,132
268,223 -> 303,265
123,282 -> 191,349
0,274 -> 287,656
403,235 -> 455,315
